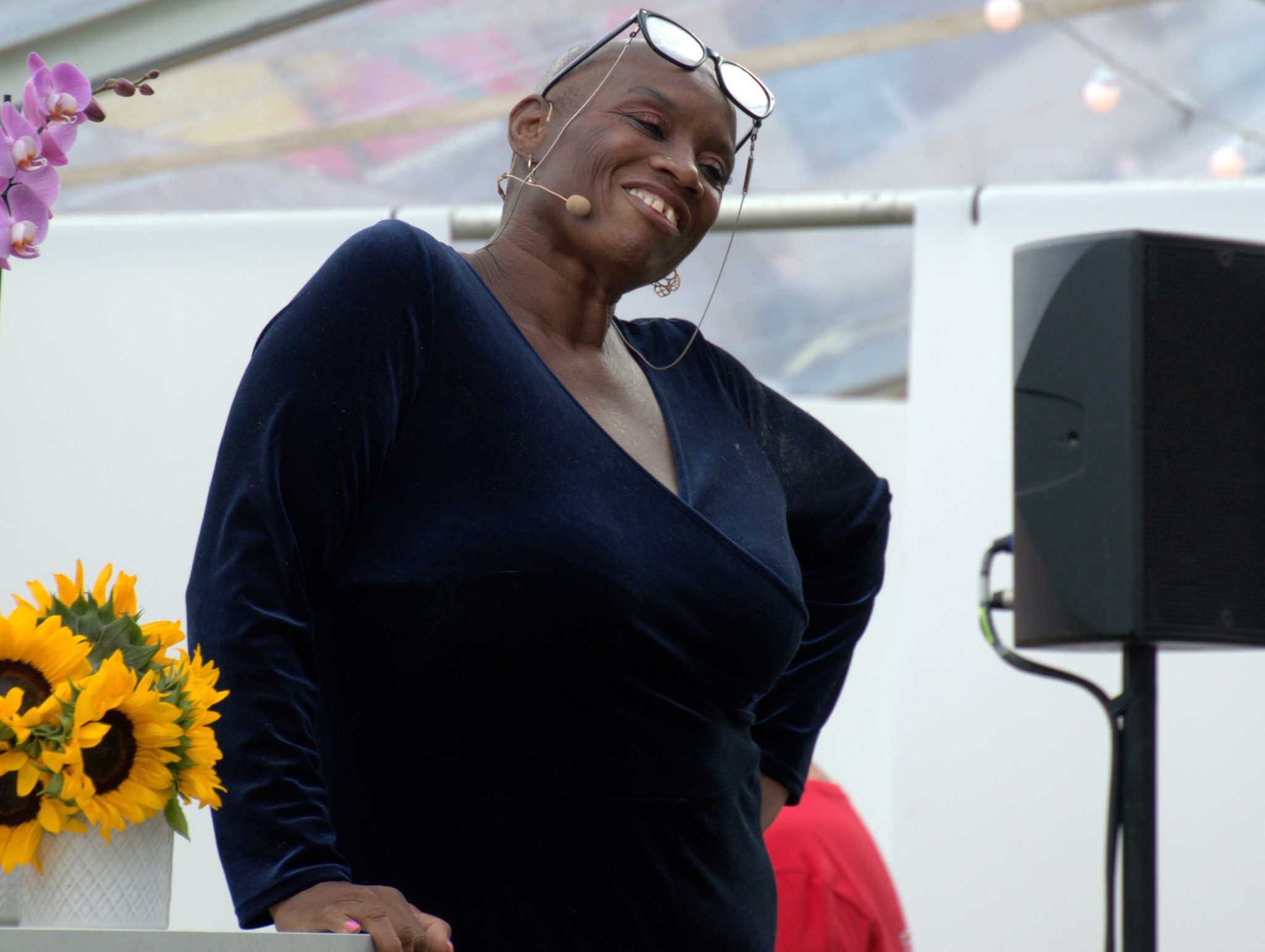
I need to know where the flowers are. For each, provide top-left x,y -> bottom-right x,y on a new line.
2,563 -> 229,872
1,50 -> 163,271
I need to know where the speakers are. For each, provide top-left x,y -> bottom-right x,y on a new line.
1012,228 -> 1264,647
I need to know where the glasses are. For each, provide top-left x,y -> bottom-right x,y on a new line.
541,8 -> 776,153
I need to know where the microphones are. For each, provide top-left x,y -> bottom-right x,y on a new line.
496,174 -> 592,218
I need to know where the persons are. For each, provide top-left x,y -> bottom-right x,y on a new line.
182,33 -> 893,952
759,758 -> 915,952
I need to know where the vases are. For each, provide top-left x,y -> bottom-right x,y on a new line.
17,815 -> 173,926
